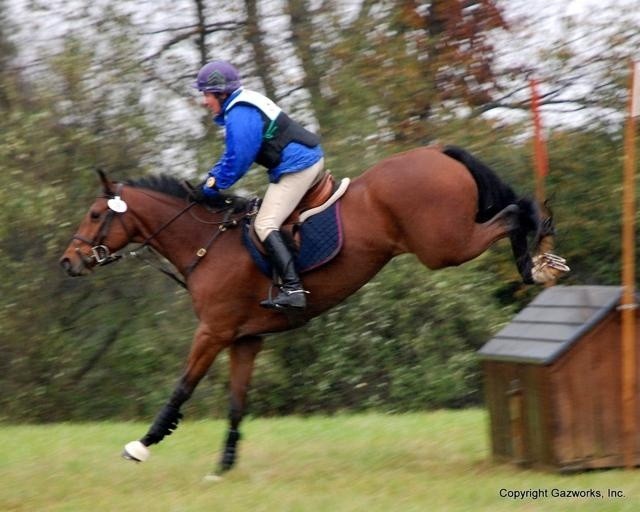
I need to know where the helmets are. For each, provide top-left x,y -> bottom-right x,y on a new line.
196,60 -> 242,95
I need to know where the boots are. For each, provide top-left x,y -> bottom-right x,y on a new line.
260,230 -> 307,312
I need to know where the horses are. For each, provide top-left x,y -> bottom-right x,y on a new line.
59,144 -> 570,481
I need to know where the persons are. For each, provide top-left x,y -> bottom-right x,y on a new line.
197,62 -> 324,308
